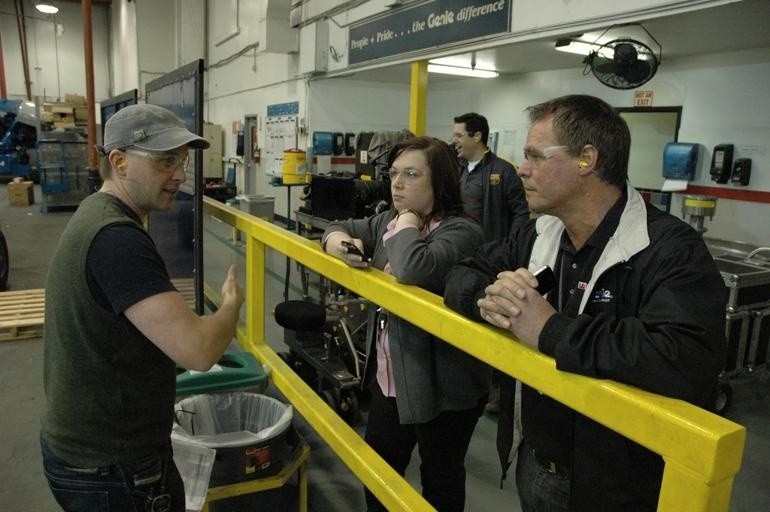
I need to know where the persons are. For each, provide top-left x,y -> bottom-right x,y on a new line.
442,94 -> 728,510
450,111 -> 530,412
321,136 -> 491,512
38,104 -> 243,512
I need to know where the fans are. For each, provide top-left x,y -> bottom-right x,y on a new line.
583,22 -> 663,90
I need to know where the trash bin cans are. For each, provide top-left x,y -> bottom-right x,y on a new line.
235,194 -> 277,249
171,351 -> 300,512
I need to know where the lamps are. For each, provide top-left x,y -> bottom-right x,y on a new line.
427,63 -> 500,79
555,39 -> 651,62
35,0 -> 59,14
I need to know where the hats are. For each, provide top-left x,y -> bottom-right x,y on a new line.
94,104 -> 210,155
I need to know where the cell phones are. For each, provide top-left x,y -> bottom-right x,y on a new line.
341,237 -> 366,265
531,263 -> 556,296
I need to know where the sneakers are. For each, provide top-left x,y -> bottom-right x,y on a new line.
485,394 -> 500,413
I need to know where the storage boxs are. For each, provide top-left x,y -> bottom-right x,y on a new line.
712,255 -> 770,377
8,181 -> 34,207
41,92 -> 88,128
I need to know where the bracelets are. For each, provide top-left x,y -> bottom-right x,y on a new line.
397,207 -> 422,229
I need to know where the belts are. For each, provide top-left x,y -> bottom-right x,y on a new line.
521,437 -> 570,475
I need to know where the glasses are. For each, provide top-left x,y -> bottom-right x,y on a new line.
388,166 -> 430,181
123,149 -> 189,172
